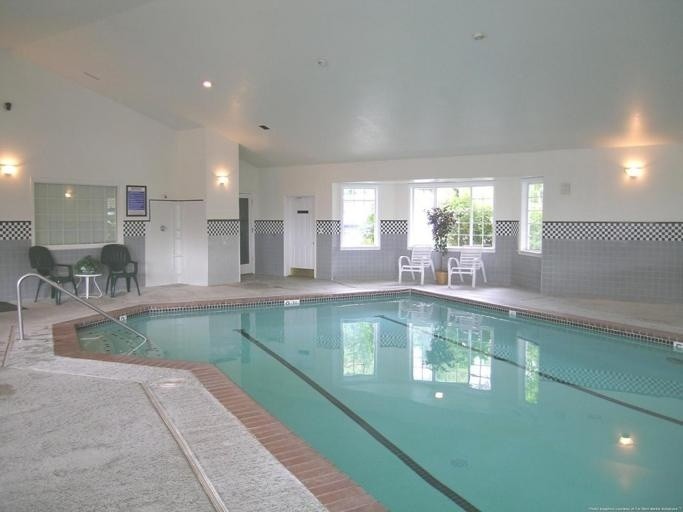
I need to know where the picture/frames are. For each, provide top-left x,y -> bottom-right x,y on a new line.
126,185 -> 147,217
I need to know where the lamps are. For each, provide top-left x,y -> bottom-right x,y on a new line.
616,430 -> 633,448
625,168 -> 642,177
218,176 -> 229,186
2,162 -> 15,175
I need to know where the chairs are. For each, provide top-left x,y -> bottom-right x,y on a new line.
101,244 -> 141,298
29,245 -> 78,306
447,246 -> 487,289
398,245 -> 436,285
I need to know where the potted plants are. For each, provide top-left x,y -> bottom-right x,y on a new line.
74,254 -> 101,274
425,205 -> 458,285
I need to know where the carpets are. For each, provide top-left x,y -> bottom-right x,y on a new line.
0,302 -> 27,312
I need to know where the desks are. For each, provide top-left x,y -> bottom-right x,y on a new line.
73,274 -> 103,299
